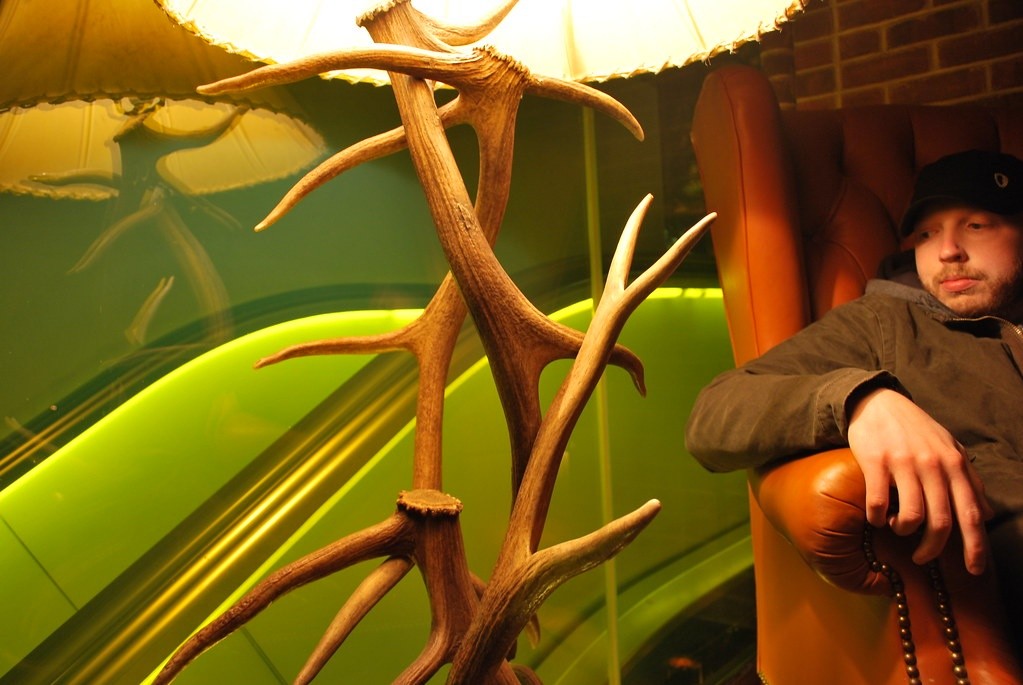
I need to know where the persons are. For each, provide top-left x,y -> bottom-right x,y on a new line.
682,147 -> 1022,685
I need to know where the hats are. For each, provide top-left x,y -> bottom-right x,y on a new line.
898,150 -> 1023,237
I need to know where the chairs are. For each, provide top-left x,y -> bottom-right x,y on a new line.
688,67 -> 1023,685
